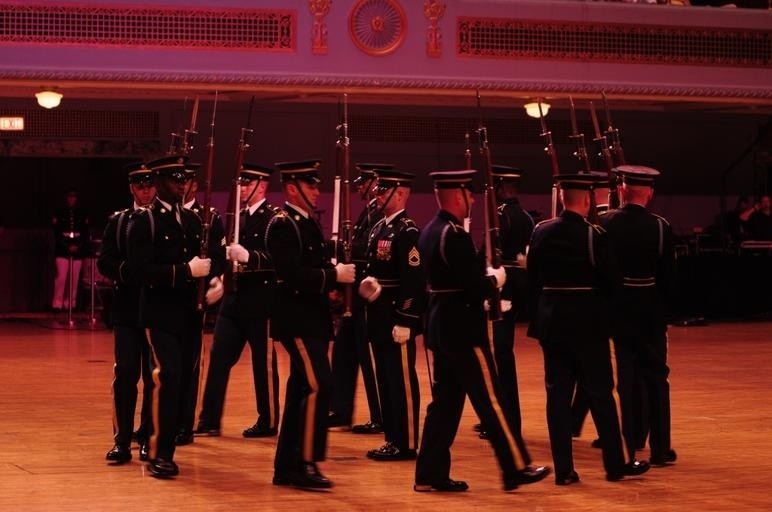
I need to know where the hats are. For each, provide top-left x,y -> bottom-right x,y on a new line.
371,168 -> 416,194
184,162 -> 202,179
240,162 -> 273,182
123,163 -> 153,186
274,159 -> 323,183
617,164 -> 662,186
145,155 -> 196,181
557,174 -> 600,190
354,162 -> 395,184
428,169 -> 482,195
491,165 -> 522,182
579,171 -> 609,186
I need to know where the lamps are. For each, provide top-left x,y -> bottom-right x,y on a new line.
34,86 -> 64,108
524,96 -> 552,118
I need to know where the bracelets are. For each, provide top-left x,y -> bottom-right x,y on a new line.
753,204 -> 760,211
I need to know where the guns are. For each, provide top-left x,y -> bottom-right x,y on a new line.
465,129 -> 472,169
166,133 -> 179,156
539,101 -> 565,220
470,86 -> 505,321
196,90 -> 217,310
340,95 -> 353,318
224,96 -> 255,293
567,96 -> 599,228
588,99 -> 621,208
331,97 -> 341,265
601,92 -> 626,166
181,93 -> 199,156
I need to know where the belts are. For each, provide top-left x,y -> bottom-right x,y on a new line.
236,264 -> 276,273
541,284 -> 591,293
65,232 -> 79,237
502,260 -> 521,268
427,286 -> 462,295
622,277 -> 656,287
377,279 -> 403,287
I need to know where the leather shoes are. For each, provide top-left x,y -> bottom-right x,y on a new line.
414,479 -> 468,491
107,443 -> 131,462
132,432 -> 143,445
242,424 -> 278,437
592,438 -> 601,447
139,440 -> 147,459
555,472 -> 578,484
366,440 -> 417,459
503,466 -> 551,491
146,456 -> 179,477
273,460 -> 330,487
175,432 -> 193,444
352,421 -> 383,434
327,414 -> 349,431
606,459 -> 648,480
650,449 -> 677,464
192,428 -> 218,436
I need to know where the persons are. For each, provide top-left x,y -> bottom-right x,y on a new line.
132,162 -> 226,445
264,160 -> 382,490
50,187 -> 90,313
739,193 -> 772,240
474,165 -> 535,440
192,161 -> 284,437
516,175 -> 650,485
127,155 -> 223,478
597,166 -> 677,467
328,164 -> 397,434
96,161 -> 155,464
569,203 -> 648,450
364,169 -> 422,461
413,170 -> 552,493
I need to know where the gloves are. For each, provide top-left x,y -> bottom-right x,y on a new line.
484,298 -> 513,313
358,276 -> 379,298
188,255 -> 211,277
335,262 -> 356,283
486,266 -> 507,288
228,243 -> 249,262
393,326 -> 410,343
206,276 -> 224,305
